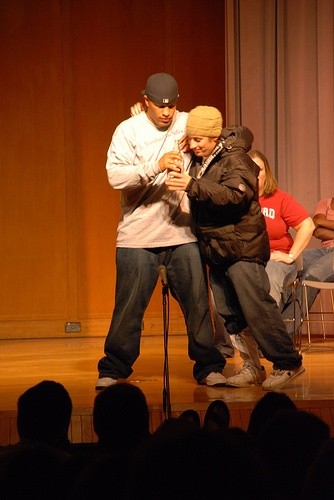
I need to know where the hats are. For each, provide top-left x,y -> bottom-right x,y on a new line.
141,73 -> 179,103
186,105 -> 223,139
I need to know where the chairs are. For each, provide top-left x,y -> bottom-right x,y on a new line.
281,252 -> 334,357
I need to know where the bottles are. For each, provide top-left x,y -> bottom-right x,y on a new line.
167,140 -> 184,179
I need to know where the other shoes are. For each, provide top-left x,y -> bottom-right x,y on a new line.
94,377 -> 117,389
200,372 -> 227,387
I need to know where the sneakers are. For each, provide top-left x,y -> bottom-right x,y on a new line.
228,363 -> 266,388
262,362 -> 306,391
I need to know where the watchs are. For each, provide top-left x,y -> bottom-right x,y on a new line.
288,252 -> 294,260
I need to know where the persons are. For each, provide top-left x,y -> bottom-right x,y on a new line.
0,380 -> 334,500
95,73 -> 227,388
131,102 -> 305,390
281,196 -> 334,334
248,150 -> 315,313
